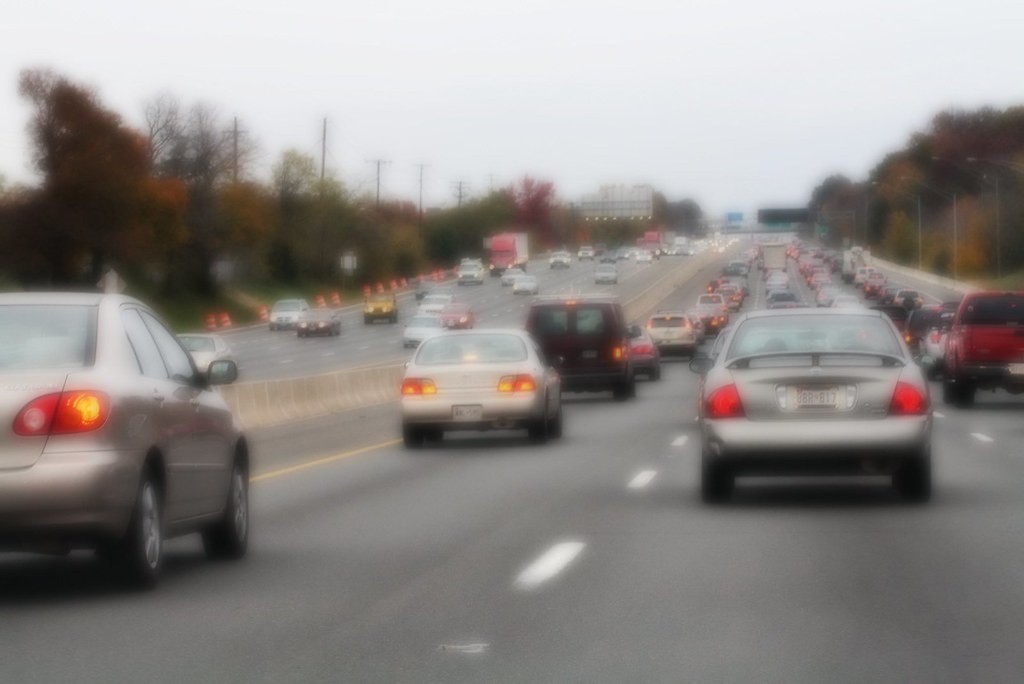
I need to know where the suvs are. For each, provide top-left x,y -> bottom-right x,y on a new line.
548,251 -> 572,270
527,297 -> 640,403
938,289 -> 1024,405
363,290 -> 398,325
647,312 -> 697,362
457,260 -> 486,286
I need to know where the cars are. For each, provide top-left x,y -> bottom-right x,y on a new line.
399,328 -> 564,451
402,313 -> 444,348
577,245 -> 595,261
499,269 -> 524,287
269,298 -> 311,331
593,264 -> 618,285
172,331 -> 234,379
440,301 -> 478,332
689,242 -> 975,382
625,324 -> 664,380
0,291 -> 251,592
295,306 -> 341,338
689,307 -> 934,503
592,228 -> 686,266
513,276 -> 539,296
414,281 -> 459,317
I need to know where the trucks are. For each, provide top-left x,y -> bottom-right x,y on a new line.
489,231 -> 528,277
